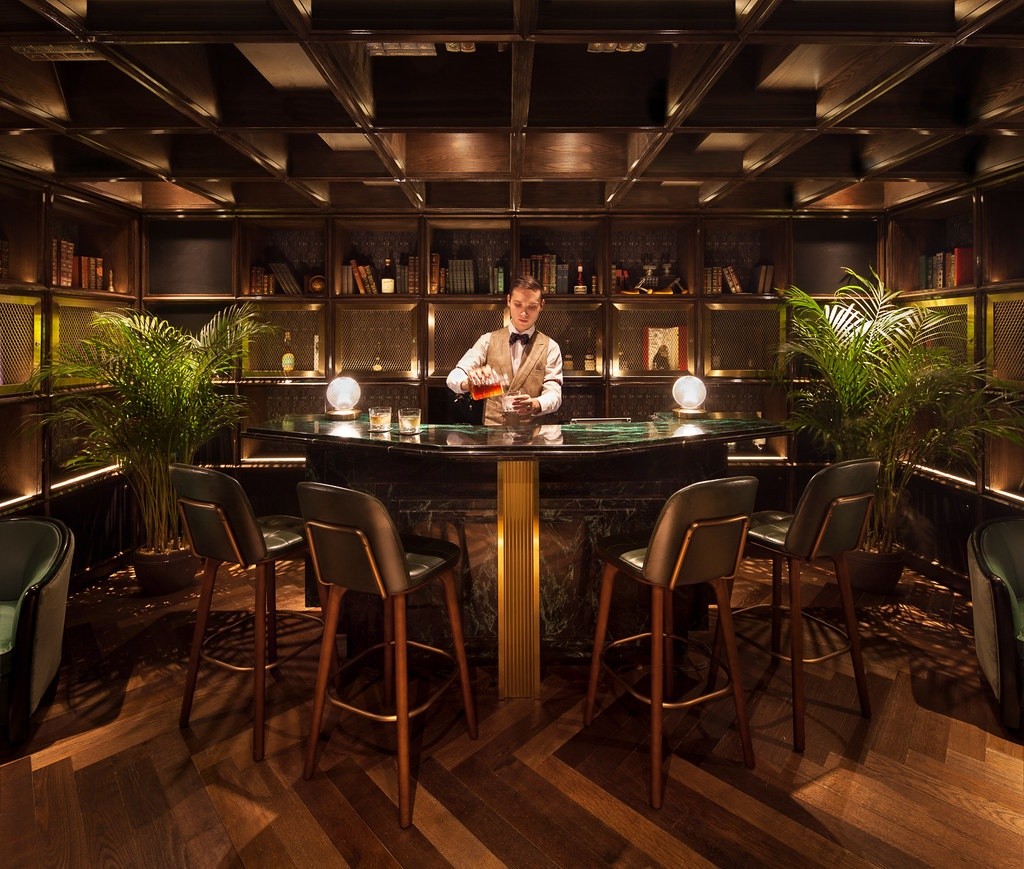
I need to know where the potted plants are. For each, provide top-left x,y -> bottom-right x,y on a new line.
773,262 -> 1024,599
15,301 -> 267,601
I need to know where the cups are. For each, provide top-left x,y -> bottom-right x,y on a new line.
502,390 -> 522,412
398,408 -> 421,434
468,367 -> 510,400
369,406 -> 391,432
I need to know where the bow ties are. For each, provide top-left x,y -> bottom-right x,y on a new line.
508,332 -> 530,345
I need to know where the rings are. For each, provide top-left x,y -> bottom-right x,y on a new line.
526,405 -> 531,410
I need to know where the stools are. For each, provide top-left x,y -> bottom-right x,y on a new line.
701,458 -> 884,754
168,463 -> 349,761
296,478 -> 486,828
584,473 -> 760,808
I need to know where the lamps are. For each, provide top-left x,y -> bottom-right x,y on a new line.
672,375 -> 708,420
326,376 -> 362,421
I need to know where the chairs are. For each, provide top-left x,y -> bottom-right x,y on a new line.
968,513 -> 1024,731
0,516 -> 77,751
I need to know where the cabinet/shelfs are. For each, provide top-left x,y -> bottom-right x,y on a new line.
0,161 -> 1024,509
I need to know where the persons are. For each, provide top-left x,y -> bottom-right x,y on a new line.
445,272 -> 564,426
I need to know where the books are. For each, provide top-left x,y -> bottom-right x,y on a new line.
0,231 -> 982,291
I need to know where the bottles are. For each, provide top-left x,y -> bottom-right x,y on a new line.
563,327 -> 573,370
573,258 -> 587,294
585,327 -> 595,370
